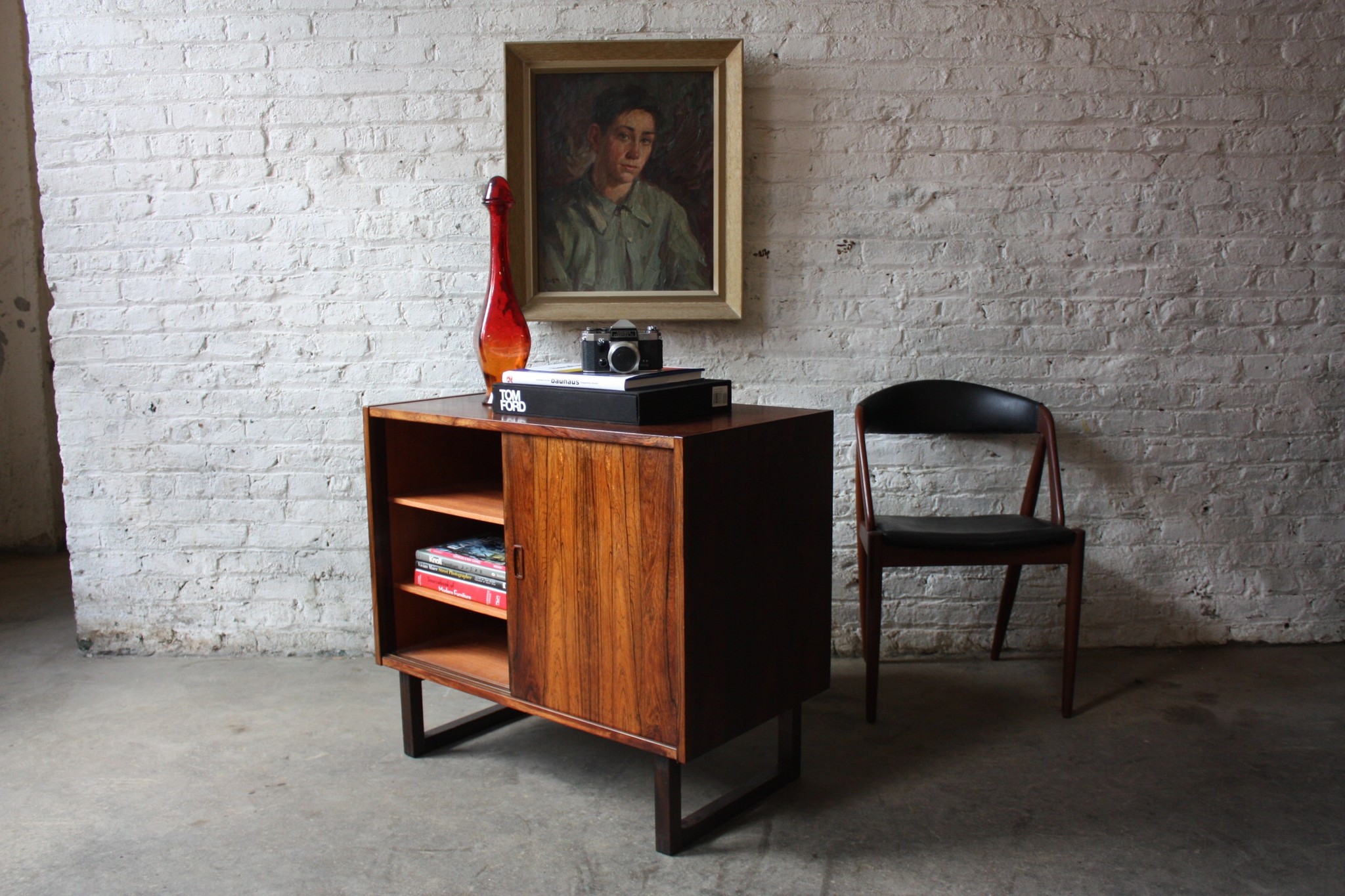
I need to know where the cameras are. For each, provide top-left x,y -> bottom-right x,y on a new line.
580,318 -> 663,374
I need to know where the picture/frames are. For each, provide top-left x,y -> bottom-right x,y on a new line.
506,38 -> 744,321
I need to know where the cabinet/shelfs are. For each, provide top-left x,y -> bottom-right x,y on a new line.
362,389 -> 834,858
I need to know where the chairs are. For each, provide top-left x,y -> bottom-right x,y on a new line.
855,378 -> 1085,721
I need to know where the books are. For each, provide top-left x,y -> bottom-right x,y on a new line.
414,536 -> 507,611
